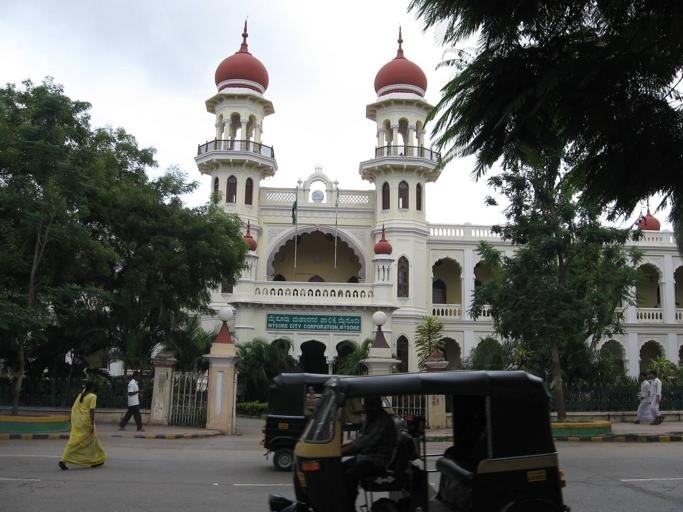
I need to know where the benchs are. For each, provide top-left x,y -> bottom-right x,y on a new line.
436,431 -> 523,512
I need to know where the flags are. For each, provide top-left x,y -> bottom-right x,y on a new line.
291,200 -> 297,225
333,188 -> 340,208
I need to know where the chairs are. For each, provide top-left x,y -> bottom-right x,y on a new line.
340,407 -> 363,433
361,432 -> 418,492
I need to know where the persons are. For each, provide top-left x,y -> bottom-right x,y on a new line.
648,369 -> 665,425
55,382 -> 105,469
631,371 -> 654,424
117,370 -> 146,432
338,393 -> 397,511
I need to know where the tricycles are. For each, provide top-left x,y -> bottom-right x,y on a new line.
262,374 -> 424,470
266,370 -> 570,512
